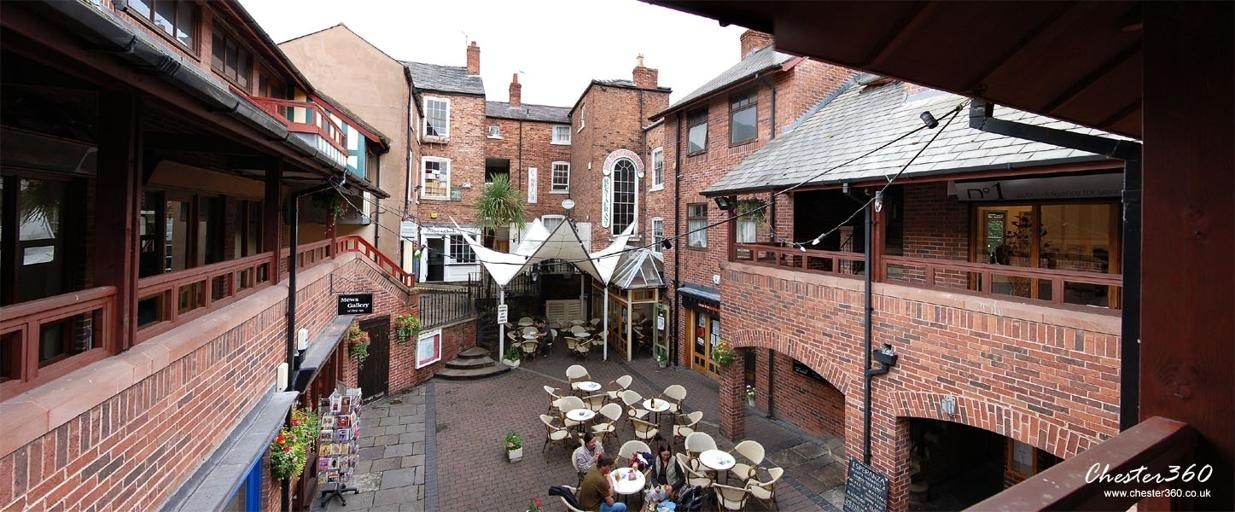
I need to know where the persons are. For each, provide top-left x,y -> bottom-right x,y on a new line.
578,454 -> 627,512
700,493 -> 720,512
535,321 -> 552,358
651,443 -> 686,497
576,431 -> 604,474
637,312 -> 648,339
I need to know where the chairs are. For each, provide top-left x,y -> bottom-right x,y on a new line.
504,311 -> 784,512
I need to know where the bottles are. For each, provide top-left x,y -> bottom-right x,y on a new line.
651,396 -> 655,409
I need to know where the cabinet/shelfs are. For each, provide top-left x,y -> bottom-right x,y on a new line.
315,391 -> 363,505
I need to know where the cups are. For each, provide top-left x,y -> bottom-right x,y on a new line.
615,473 -> 621,482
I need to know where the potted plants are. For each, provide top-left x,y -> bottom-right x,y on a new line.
470,172 -> 528,241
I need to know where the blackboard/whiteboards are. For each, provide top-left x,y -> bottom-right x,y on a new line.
843,456 -> 889,512
792,358 -> 828,386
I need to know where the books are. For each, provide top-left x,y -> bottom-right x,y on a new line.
320,395 -> 360,482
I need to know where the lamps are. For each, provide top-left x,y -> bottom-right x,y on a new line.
294,327 -> 309,370
712,193 -> 731,211
661,237 -> 673,250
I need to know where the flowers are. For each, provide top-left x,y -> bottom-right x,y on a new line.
394,313 -> 421,341
273,424 -> 310,484
287,404 -> 321,443
350,325 -> 371,364
